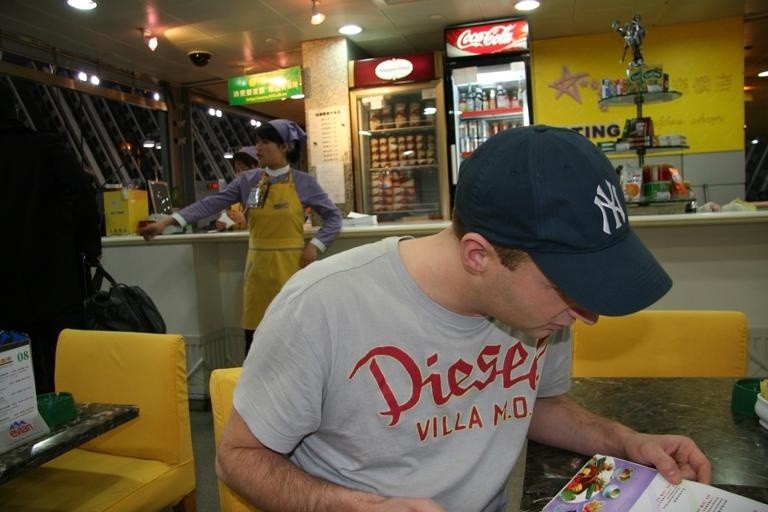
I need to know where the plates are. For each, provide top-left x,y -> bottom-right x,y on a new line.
603,484 -> 615,497
559,454 -> 615,504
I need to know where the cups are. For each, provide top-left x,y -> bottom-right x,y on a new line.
606,485 -> 621,500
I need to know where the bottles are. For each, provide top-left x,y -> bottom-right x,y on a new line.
457,83 -> 521,112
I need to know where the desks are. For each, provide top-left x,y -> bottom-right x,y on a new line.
520,370 -> 761,512
10,399 -> 140,479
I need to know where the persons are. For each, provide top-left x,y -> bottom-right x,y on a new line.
0,76 -> 107,395
620,13 -> 646,64
214,125 -> 712,512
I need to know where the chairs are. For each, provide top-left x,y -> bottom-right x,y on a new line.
209,368 -> 263,512
556,308 -> 746,385
10,327 -> 197,512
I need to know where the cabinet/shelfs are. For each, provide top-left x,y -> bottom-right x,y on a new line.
598,92 -> 692,210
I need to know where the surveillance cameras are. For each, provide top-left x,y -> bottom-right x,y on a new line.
187,50 -> 213,68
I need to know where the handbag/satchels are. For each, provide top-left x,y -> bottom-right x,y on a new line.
81,258 -> 166,333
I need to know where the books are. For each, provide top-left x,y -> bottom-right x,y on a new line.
597,118 -> 686,152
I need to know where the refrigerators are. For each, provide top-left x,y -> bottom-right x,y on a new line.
348,49 -> 445,223
443,14 -> 538,222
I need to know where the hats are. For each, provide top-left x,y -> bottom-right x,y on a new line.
454,124 -> 673,317
